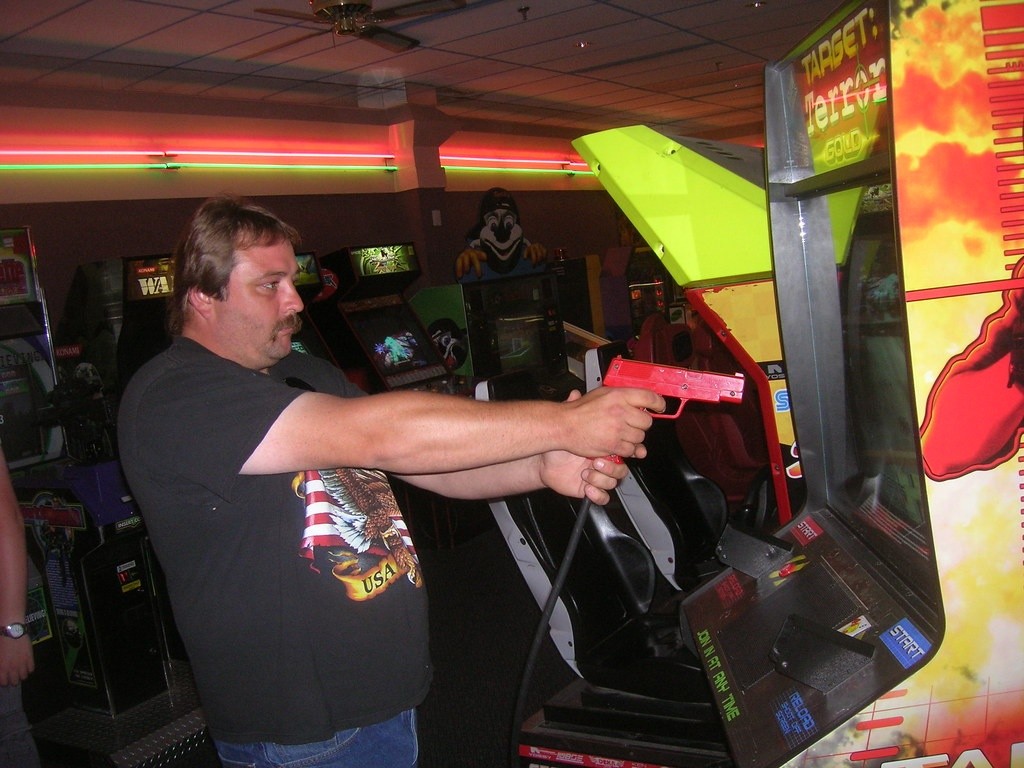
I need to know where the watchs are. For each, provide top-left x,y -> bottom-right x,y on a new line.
0,623 -> 27,639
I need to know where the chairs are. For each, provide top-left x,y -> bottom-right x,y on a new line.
472,363 -> 721,723
633,313 -> 769,507
691,317 -> 799,469
579,340 -> 730,594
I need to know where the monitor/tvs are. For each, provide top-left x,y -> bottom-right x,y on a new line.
487,307 -> 567,383
346,297 -> 454,389
0,358 -> 43,469
837,194 -> 947,549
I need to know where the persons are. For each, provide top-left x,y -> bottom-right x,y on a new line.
114,190 -> 667,767
0,447 -> 41,768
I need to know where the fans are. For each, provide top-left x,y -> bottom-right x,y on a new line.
233,0 -> 467,64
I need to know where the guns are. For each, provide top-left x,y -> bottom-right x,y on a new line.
593,355 -> 746,465
